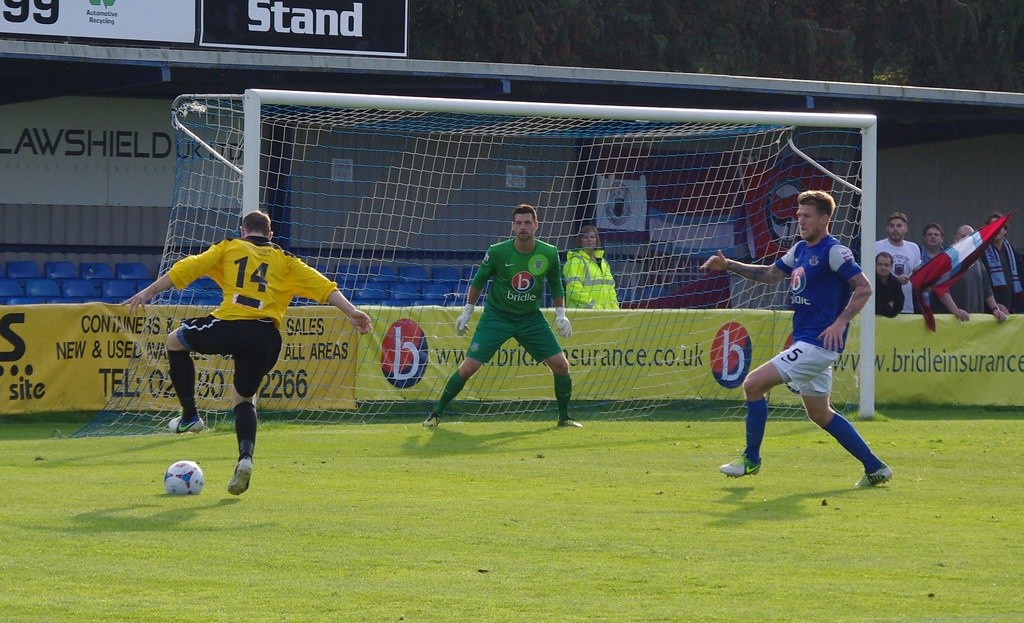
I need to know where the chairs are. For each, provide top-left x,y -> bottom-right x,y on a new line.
0,260 -> 669,307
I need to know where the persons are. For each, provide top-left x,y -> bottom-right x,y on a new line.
119,209 -> 374,496
699,188 -> 895,491
422,203 -> 585,429
562,225 -> 620,310
875,211 -> 1024,324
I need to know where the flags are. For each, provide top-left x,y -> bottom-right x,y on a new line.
907,207 -> 1021,333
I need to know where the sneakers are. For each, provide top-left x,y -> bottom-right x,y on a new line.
556,416 -> 582,427
854,462 -> 892,487
168,413 -> 205,434
422,411 -> 441,426
227,457 -> 252,495
719,452 -> 762,479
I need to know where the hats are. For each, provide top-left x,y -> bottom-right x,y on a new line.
887,211 -> 908,223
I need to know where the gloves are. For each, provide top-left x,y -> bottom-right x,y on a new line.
555,305 -> 573,340
455,304 -> 475,336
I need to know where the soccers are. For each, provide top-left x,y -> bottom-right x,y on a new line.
165,460 -> 205,495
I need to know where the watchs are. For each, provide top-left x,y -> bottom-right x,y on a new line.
989,306 -> 999,314
